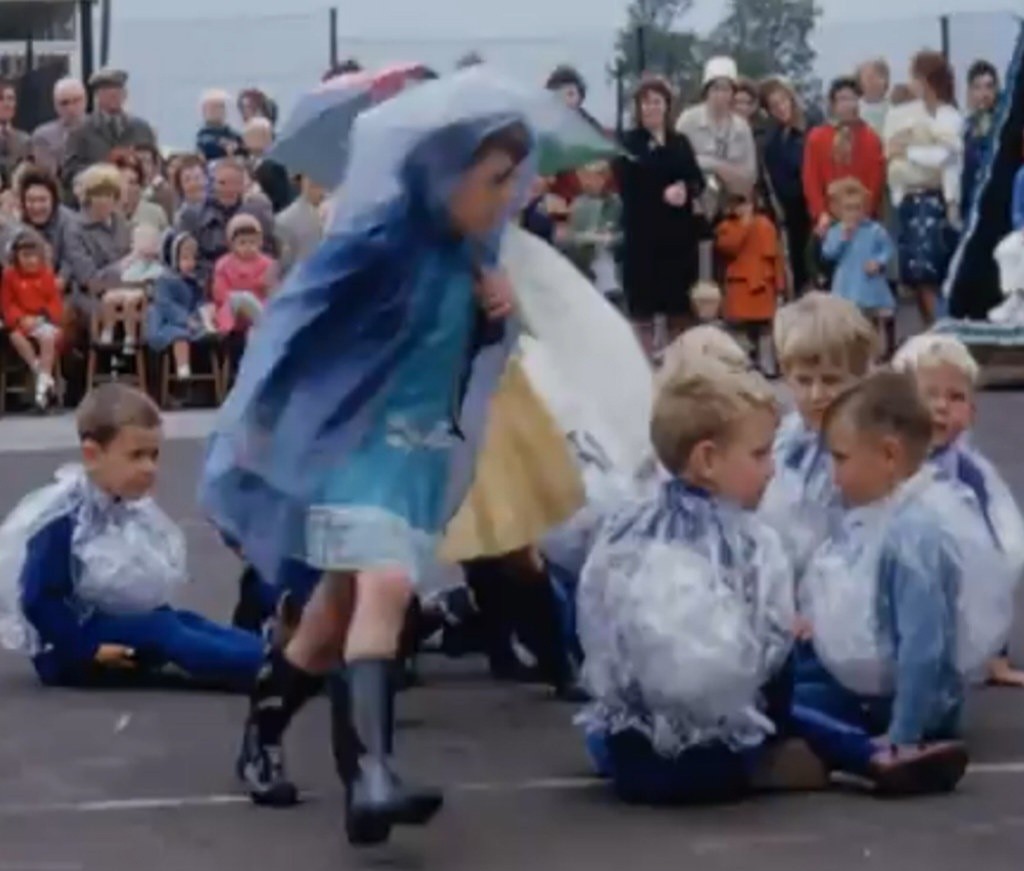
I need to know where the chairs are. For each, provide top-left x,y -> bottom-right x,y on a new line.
0,280 -> 232,410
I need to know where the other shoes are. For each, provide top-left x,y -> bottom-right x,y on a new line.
611,728 -> 736,804
744,738 -> 826,789
35,367 -> 56,408
873,738 -> 969,795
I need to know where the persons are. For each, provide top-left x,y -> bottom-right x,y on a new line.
199,72 -> 538,844
573,293 -> 1024,796
0,345 -> 591,701
0,61 -> 358,408
519,47 -> 1024,377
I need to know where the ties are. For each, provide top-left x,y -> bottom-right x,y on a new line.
111,117 -> 118,139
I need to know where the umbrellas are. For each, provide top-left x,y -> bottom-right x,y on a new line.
531,94 -> 637,174
263,61 -> 429,195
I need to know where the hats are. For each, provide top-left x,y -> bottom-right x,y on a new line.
701,56 -> 740,87
81,163 -> 121,202
228,216 -> 264,244
88,69 -> 127,87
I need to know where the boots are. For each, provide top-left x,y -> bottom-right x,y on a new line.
331,659 -> 445,845
474,580 -> 533,683
506,555 -> 583,695
238,644 -> 325,803
